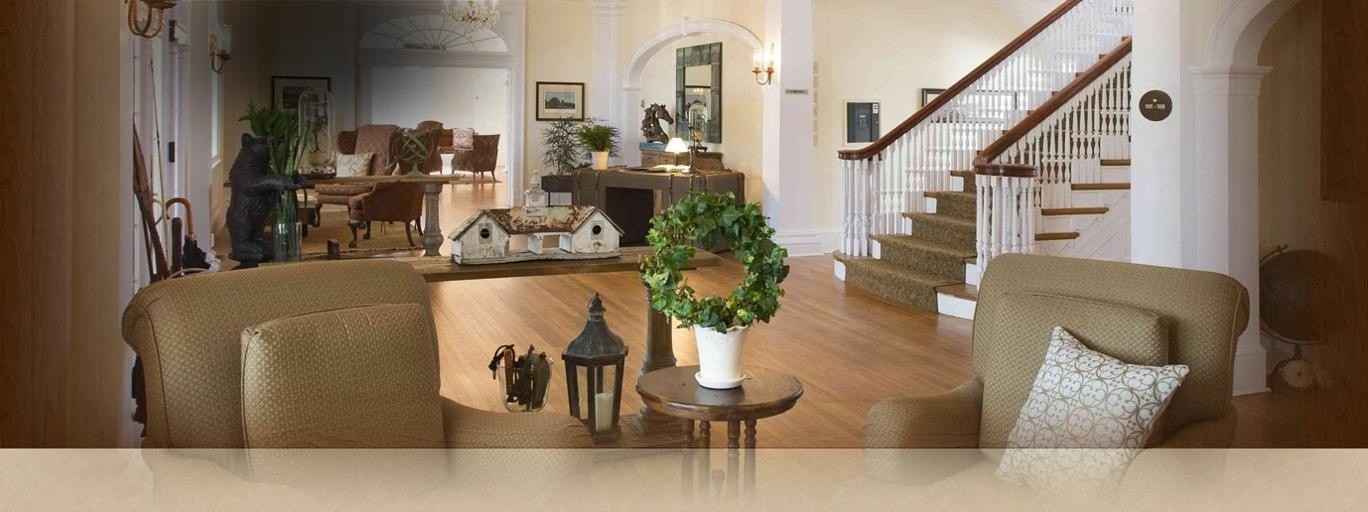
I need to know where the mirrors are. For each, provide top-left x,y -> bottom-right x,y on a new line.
674,40 -> 725,147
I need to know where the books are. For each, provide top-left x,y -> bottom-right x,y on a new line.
648,164 -> 690,173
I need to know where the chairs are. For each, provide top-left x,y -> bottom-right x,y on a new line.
312,118 -> 406,239
117,255 -> 598,509
345,125 -> 443,249
450,130 -> 504,181
408,120 -> 446,176
825,252 -> 1249,511
288,124 -> 357,228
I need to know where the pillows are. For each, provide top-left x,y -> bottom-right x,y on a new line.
996,323 -> 1192,498
391,161 -> 405,181
333,150 -> 376,181
452,127 -> 475,153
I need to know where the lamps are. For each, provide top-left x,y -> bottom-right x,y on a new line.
125,0 -> 178,40
441,0 -> 508,40
207,18 -> 234,77
560,287 -> 623,449
750,43 -> 777,88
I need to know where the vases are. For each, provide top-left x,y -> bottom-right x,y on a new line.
540,176 -> 574,195
631,182 -> 794,391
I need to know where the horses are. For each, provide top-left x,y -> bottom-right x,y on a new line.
639,102 -> 675,145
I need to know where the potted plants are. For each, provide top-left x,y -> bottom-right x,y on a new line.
239,85 -> 329,262
577,125 -> 619,175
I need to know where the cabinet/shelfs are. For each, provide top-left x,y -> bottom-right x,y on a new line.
571,148 -> 747,255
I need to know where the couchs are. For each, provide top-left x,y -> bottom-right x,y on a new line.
437,126 -> 478,154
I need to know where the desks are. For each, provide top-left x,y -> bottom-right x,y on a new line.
293,171 -> 466,260
581,405 -> 702,511
632,364 -> 805,509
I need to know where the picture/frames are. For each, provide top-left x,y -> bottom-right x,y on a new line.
534,80 -> 585,125
920,86 -> 1019,126
682,83 -> 712,123
269,72 -> 334,127
841,98 -> 884,150
